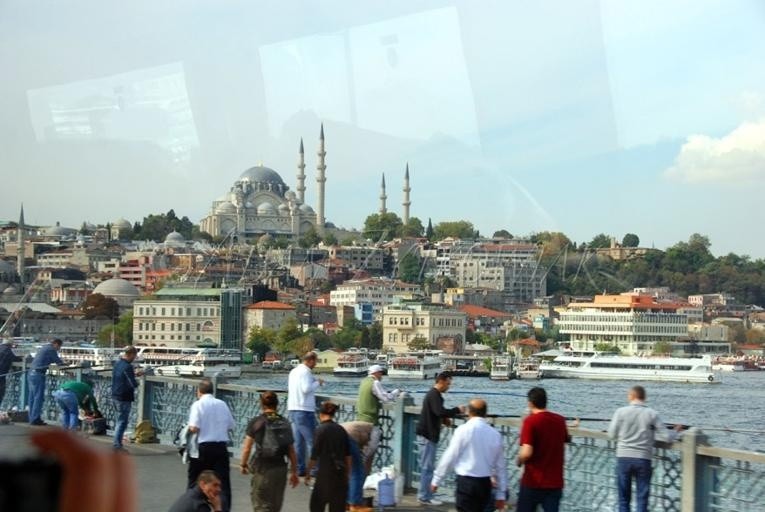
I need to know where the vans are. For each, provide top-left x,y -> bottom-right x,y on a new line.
263,361 -> 273,369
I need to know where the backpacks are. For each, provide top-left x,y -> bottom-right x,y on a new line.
259,414 -> 294,462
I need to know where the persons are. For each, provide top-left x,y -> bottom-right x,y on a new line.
240,392 -> 300,512
430,399 -> 506,512
0,338 -> 22,407
608,385 -> 682,512
415,371 -> 466,505
111,346 -> 139,452
55,381 -> 100,432
27,339 -> 70,425
339,420 -> 381,512
287,351 -> 325,476
187,380 -> 236,512
305,403 -> 350,512
354,364 -> 402,425
168,470 -> 222,512
516,387 -> 570,512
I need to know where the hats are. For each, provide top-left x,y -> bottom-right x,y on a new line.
367,365 -> 384,374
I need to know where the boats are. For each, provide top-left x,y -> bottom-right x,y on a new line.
713,356 -> 764,371
29,346 -> 114,371
134,349 -> 241,379
490,355 -> 512,380
515,362 -> 540,379
539,352 -> 715,383
333,347 -> 368,377
388,357 -> 441,379
443,356 -> 490,375
